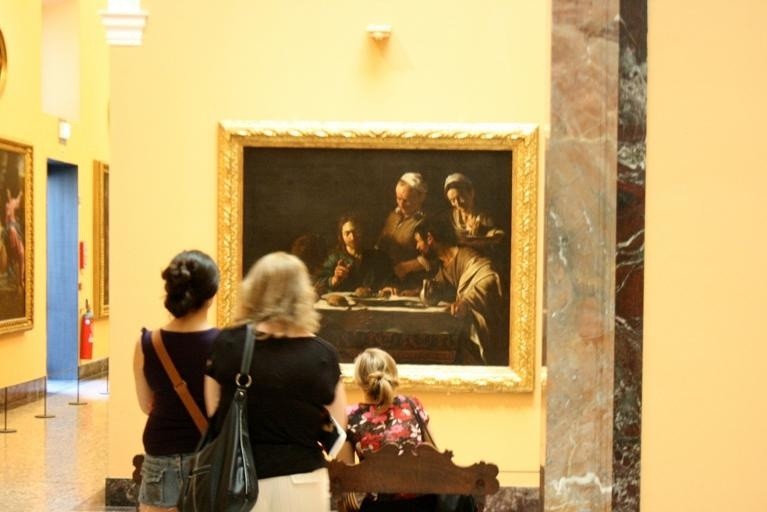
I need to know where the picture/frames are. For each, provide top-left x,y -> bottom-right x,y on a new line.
218,124 -> 539,395
0,138 -> 34,334
92,158 -> 109,323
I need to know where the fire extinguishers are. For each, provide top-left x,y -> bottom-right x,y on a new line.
80,300 -> 95,360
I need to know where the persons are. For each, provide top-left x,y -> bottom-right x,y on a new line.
203,250 -> 341,512
134,250 -> 223,512
315,171 -> 507,363
0,187 -> 25,294
337,348 -> 439,512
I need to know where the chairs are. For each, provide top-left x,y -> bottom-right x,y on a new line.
316,441 -> 500,512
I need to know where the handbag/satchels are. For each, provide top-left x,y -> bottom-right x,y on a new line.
177,324 -> 259,512
405,397 -> 475,512
